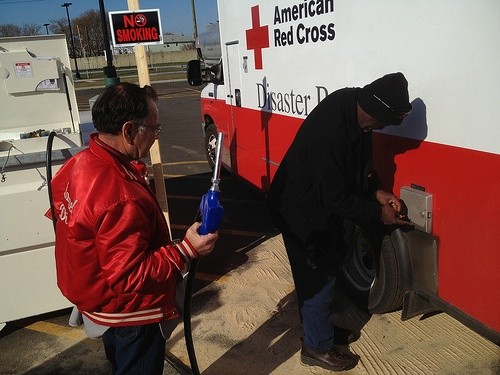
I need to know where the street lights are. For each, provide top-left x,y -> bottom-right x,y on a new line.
41,23 -> 52,36
60,2 -> 81,80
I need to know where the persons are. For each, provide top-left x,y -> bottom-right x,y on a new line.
50,81 -> 220,375
269,71 -> 414,371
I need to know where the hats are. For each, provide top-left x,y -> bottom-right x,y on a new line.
358,72 -> 412,126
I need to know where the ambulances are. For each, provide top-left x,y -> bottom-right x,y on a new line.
186,0 -> 498,336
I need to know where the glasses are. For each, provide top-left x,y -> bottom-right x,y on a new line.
132,123 -> 162,136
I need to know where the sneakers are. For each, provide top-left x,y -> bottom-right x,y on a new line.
300,337 -> 358,371
333,325 -> 360,345
